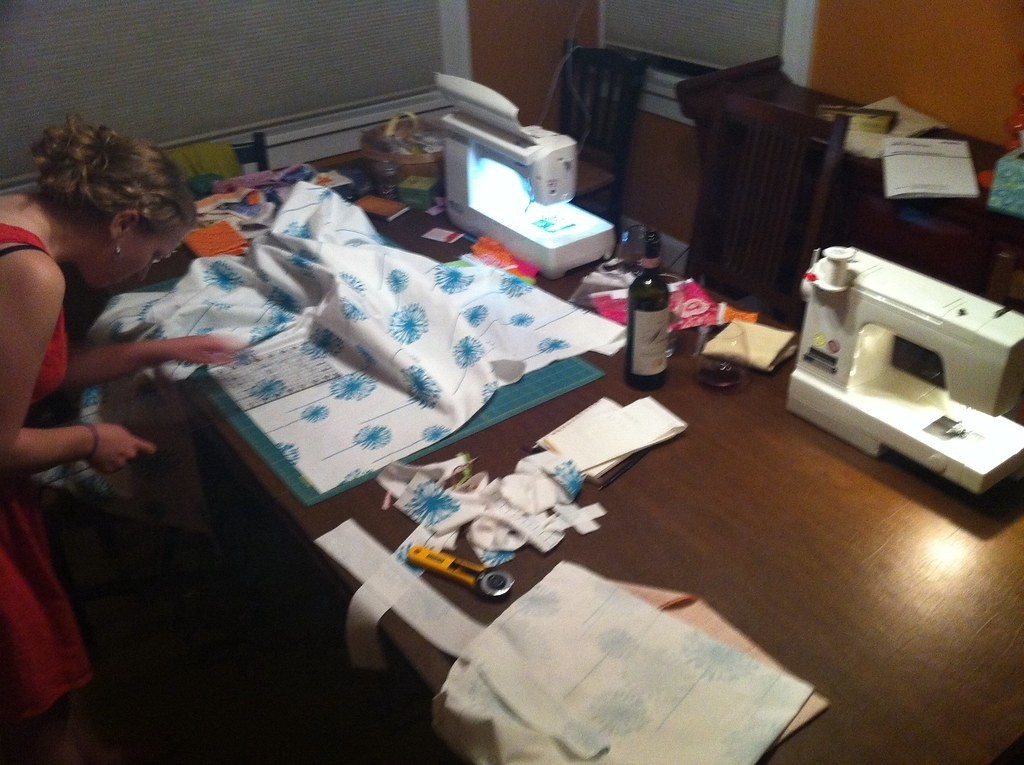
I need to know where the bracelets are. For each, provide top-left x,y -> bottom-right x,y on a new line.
81,422 -> 98,459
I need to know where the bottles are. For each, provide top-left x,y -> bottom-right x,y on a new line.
624,231 -> 669,390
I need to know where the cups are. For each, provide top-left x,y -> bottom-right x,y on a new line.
658,273 -> 685,358
697,320 -> 748,388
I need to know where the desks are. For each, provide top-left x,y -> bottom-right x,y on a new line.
109,115 -> 1024,765
684,53 -> 1024,291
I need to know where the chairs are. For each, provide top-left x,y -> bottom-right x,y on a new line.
556,43 -> 644,238
688,88 -> 852,329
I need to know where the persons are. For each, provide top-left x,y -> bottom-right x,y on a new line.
0,113 -> 233,765
